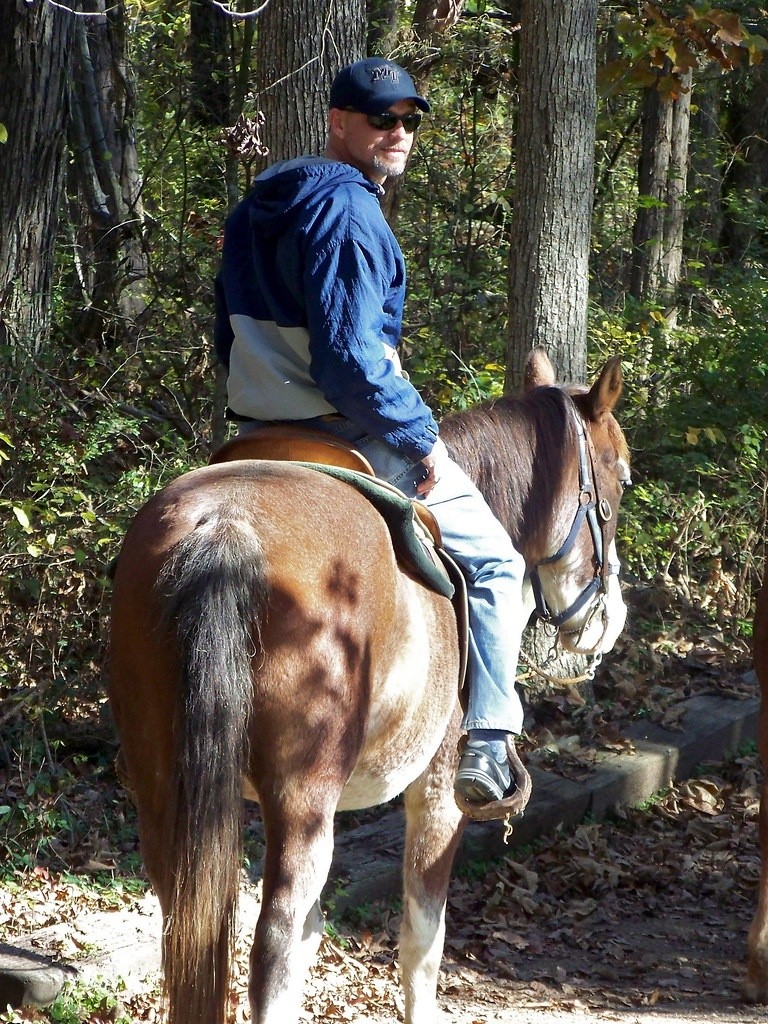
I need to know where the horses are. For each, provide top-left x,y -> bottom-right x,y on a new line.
103,345 -> 634,1024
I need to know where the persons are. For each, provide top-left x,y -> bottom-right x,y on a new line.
211,58 -> 529,803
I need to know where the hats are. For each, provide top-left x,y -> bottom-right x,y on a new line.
328,56 -> 433,117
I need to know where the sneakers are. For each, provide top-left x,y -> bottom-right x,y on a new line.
456,739 -> 515,805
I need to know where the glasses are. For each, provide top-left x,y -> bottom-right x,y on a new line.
338,103 -> 422,132
